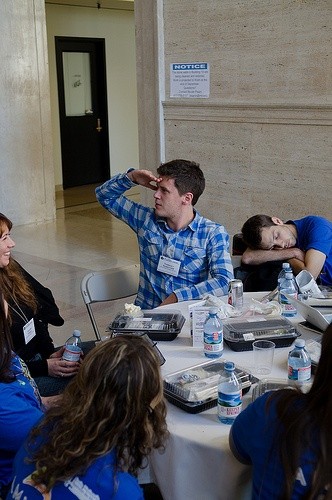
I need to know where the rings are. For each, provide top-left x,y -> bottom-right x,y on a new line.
61,373 -> 64,377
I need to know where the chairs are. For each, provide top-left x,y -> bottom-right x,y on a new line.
80,264 -> 140,341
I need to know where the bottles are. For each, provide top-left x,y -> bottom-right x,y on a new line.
217,362 -> 242,425
203,308 -> 224,358
59,330 -> 83,374
278,263 -> 298,317
287,339 -> 311,384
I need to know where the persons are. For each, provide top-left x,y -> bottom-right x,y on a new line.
228,318 -> 332,500
95,159 -> 235,310
0,290 -> 64,452
0,213 -> 100,396
241,215 -> 332,286
8,335 -> 169,500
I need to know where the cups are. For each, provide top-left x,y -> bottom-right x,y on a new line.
252,340 -> 275,375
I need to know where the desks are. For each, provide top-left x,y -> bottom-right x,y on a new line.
122,291 -> 332,499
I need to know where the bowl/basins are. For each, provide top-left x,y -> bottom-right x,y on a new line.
161,359 -> 260,414
221,316 -> 303,352
105,309 -> 186,341
251,378 -> 312,403
288,334 -> 323,374
